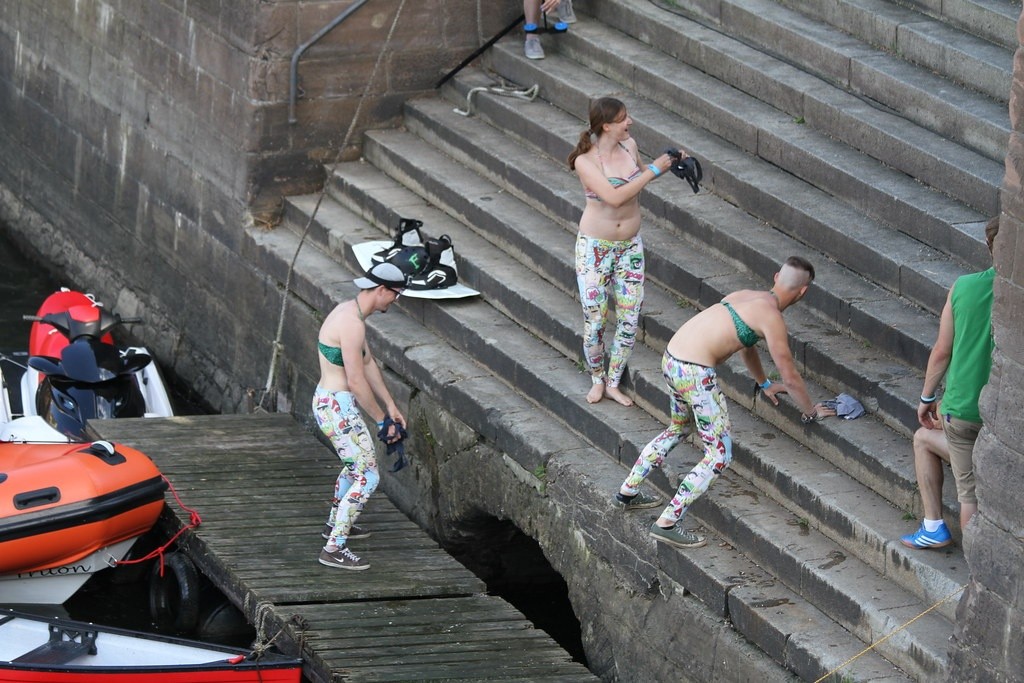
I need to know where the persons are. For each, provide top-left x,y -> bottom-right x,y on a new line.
610,256 -> 836,549
313,263 -> 408,571
917,214 -> 1002,530
523,0 -> 577,61
574,97 -> 690,408
901,406 -> 952,548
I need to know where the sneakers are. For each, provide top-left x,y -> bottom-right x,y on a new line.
408,234 -> 458,289
371,218 -> 423,265
320,523 -> 371,539
611,491 -> 663,508
899,523 -> 951,549
525,36 -> 546,60
557,0 -> 577,24
649,523 -> 707,549
319,546 -> 371,570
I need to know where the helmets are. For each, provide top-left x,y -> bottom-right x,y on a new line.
391,246 -> 429,276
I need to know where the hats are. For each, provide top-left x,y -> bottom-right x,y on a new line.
353,262 -> 406,289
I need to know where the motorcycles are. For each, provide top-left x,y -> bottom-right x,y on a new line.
0,287 -> 174,445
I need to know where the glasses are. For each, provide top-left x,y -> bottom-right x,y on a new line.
389,287 -> 401,299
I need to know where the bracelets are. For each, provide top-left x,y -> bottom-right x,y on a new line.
759,378 -> 772,390
648,164 -> 661,177
377,421 -> 384,426
920,392 -> 937,405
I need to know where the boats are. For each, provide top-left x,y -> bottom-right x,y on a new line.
1,608 -> 303,683
1,439 -> 169,605
